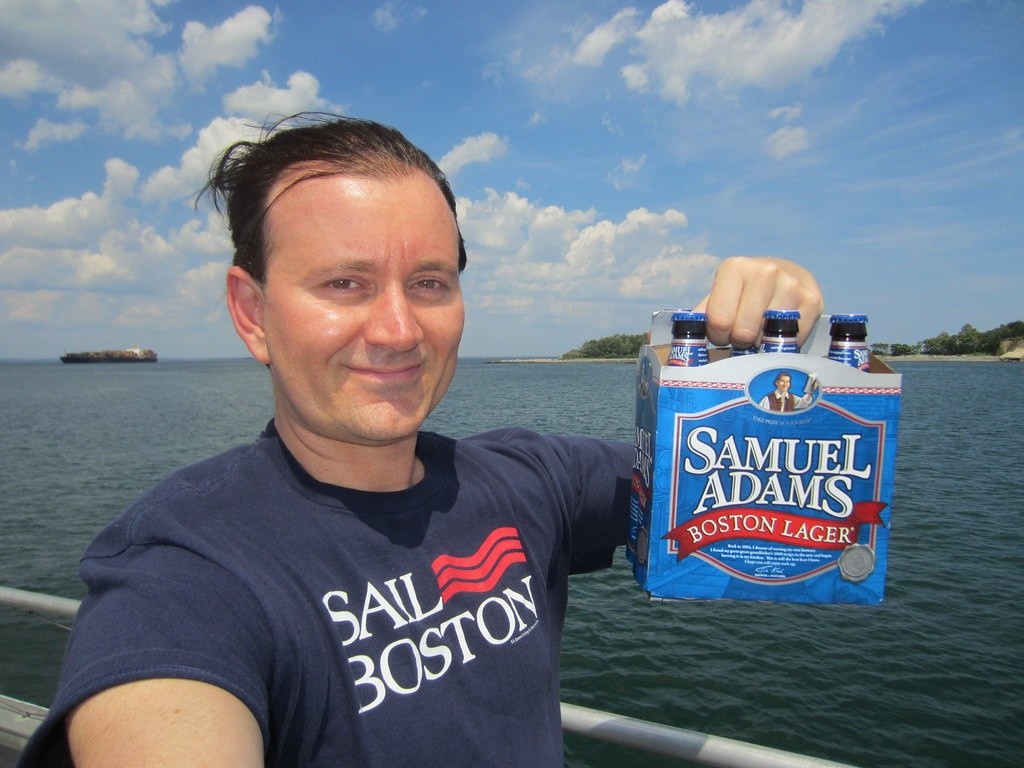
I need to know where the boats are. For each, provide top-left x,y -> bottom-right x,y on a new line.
59,344 -> 158,363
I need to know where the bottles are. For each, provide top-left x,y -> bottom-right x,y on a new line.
662,310 -> 709,368
761,309 -> 803,355
827,314 -> 872,373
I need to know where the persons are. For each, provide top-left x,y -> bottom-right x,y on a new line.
11,110 -> 826,766
758,372 -> 818,413
